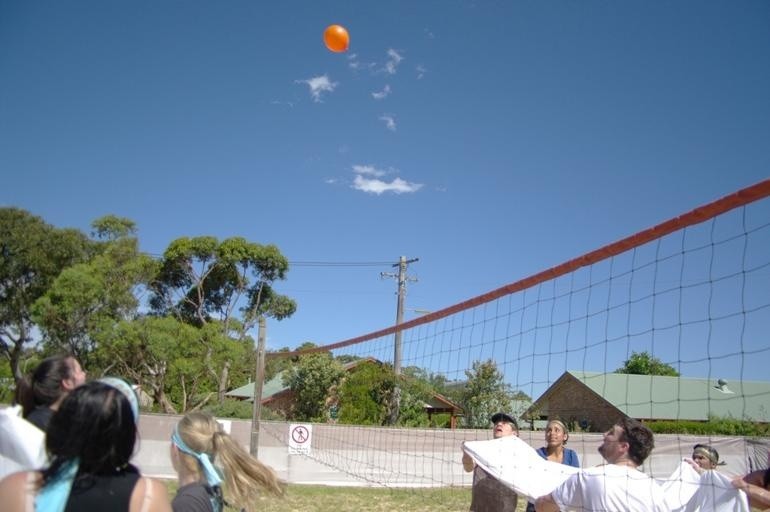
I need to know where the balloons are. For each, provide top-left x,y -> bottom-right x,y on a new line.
323,24 -> 350,52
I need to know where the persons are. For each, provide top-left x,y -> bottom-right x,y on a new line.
2,377 -> 174,512
535,415 -> 671,511
684,445 -> 769,512
18,355 -> 86,431
169,411 -> 289,512
526,416 -> 579,511
461,412 -> 518,511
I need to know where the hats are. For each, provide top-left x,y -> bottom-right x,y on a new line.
492,413 -> 517,427
